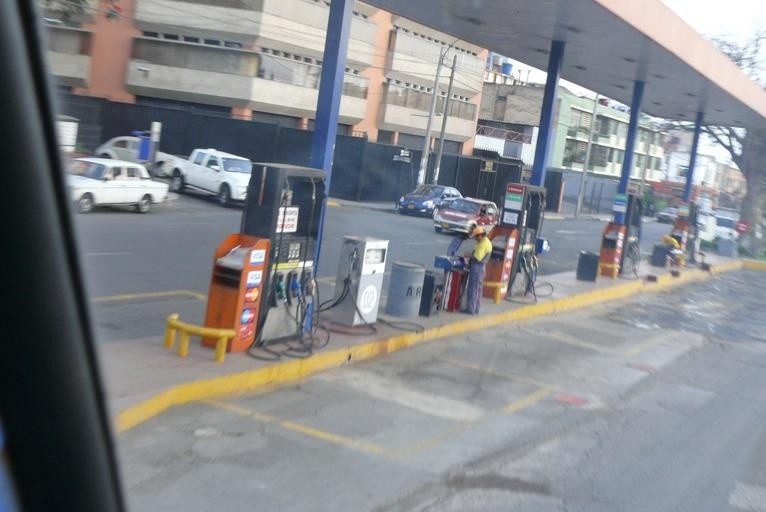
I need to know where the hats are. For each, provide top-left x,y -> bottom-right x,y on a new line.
469,225 -> 485,239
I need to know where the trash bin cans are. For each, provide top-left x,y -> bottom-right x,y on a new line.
652,244 -> 670,266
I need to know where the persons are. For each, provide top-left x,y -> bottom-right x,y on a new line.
661,235 -> 681,249
460,226 -> 492,315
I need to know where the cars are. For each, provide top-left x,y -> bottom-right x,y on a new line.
62,135 -> 252,214
714,215 -> 740,241
656,207 -> 678,224
399,183 -> 500,239
